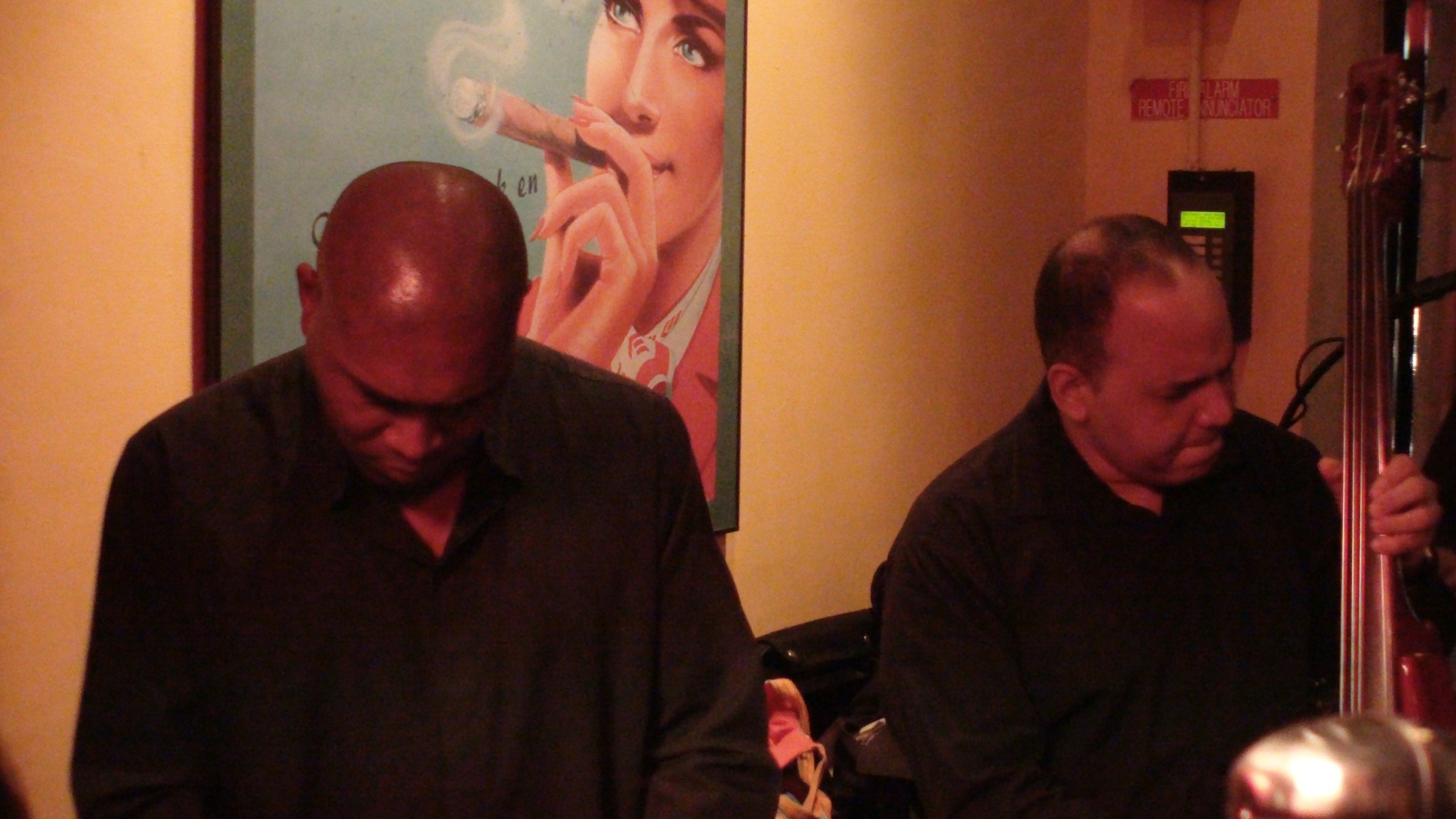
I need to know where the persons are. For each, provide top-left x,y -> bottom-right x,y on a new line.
68,161 -> 779,819
865,214 -> 1438,819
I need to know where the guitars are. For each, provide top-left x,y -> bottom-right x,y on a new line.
1339,56 -> 1456,762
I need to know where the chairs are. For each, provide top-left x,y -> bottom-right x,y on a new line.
754,607 -> 919,819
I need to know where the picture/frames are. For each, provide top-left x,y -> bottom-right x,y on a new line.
192,1 -> 748,536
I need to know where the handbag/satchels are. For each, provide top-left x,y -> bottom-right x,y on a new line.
763,679 -> 837,819
